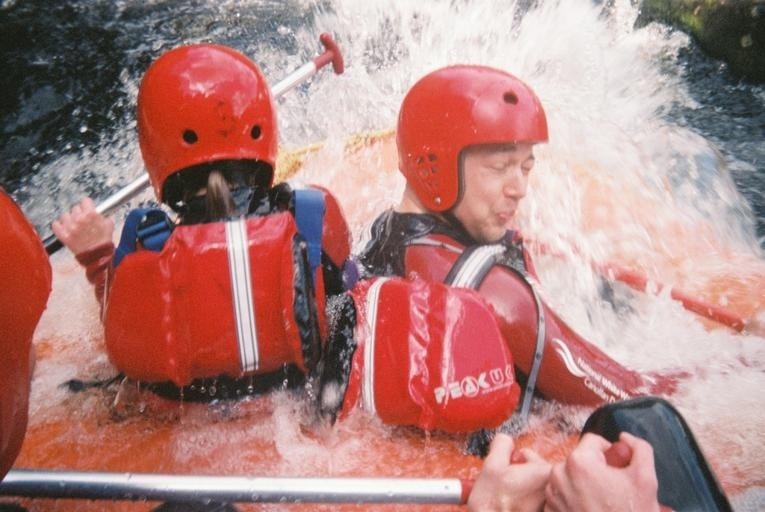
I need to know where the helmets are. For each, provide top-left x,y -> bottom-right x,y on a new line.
132,40 -> 279,208
393,63 -> 552,214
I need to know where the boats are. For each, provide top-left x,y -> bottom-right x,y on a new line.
0,136 -> 765,510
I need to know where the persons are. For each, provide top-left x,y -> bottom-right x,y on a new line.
545,432 -> 660,512
464,432 -> 552,512
336,63 -> 764,436
49,43 -> 352,420
